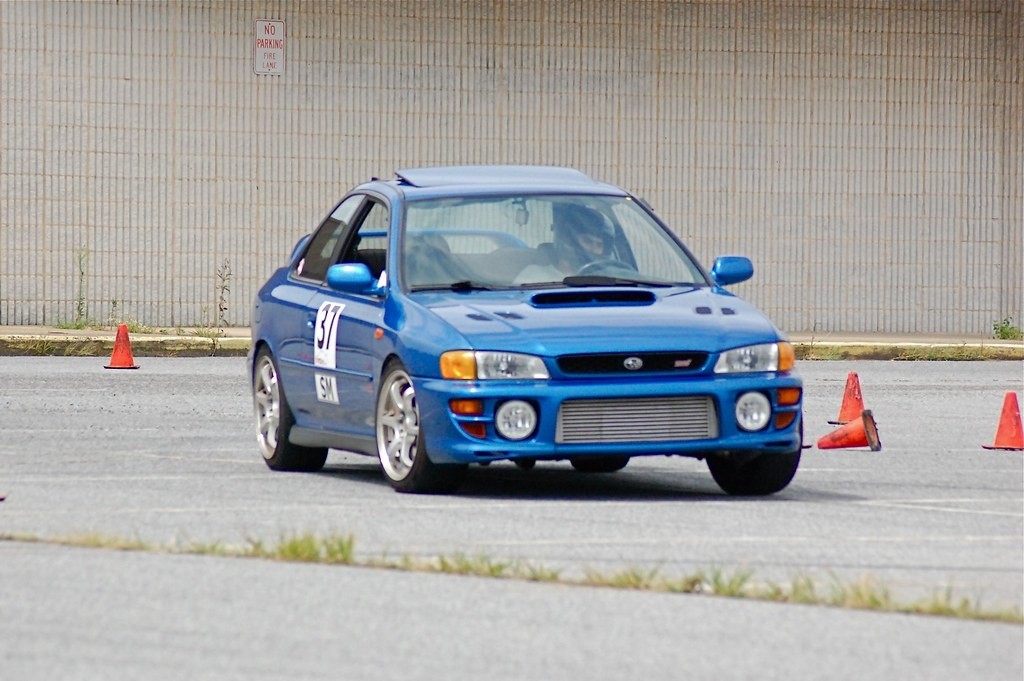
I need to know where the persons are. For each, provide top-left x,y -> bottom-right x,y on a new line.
513,206 -> 615,285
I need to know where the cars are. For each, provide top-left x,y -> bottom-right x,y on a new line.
248,163 -> 805,495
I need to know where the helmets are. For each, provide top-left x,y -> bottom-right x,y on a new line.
553,207 -> 615,263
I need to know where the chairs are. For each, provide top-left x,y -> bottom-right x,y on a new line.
406,233 -> 469,286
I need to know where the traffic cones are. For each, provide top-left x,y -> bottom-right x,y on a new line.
102,325 -> 140,369
818,409 -> 881,452
980,390 -> 1024,450
827,371 -> 866,425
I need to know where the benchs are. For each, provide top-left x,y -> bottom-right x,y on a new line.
350,246 -> 530,281
537,244 -> 557,264
